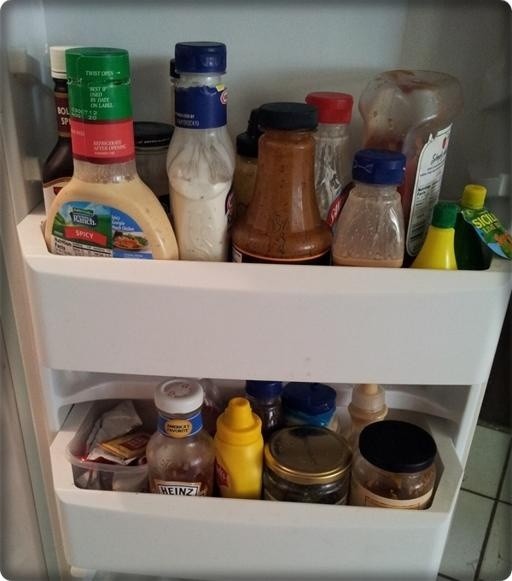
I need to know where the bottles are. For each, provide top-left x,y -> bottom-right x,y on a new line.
39,39 -> 488,271
144,377 -> 439,510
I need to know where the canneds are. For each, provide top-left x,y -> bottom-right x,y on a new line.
351,420 -> 437,508
265,425 -> 352,505
131,121 -> 175,217
